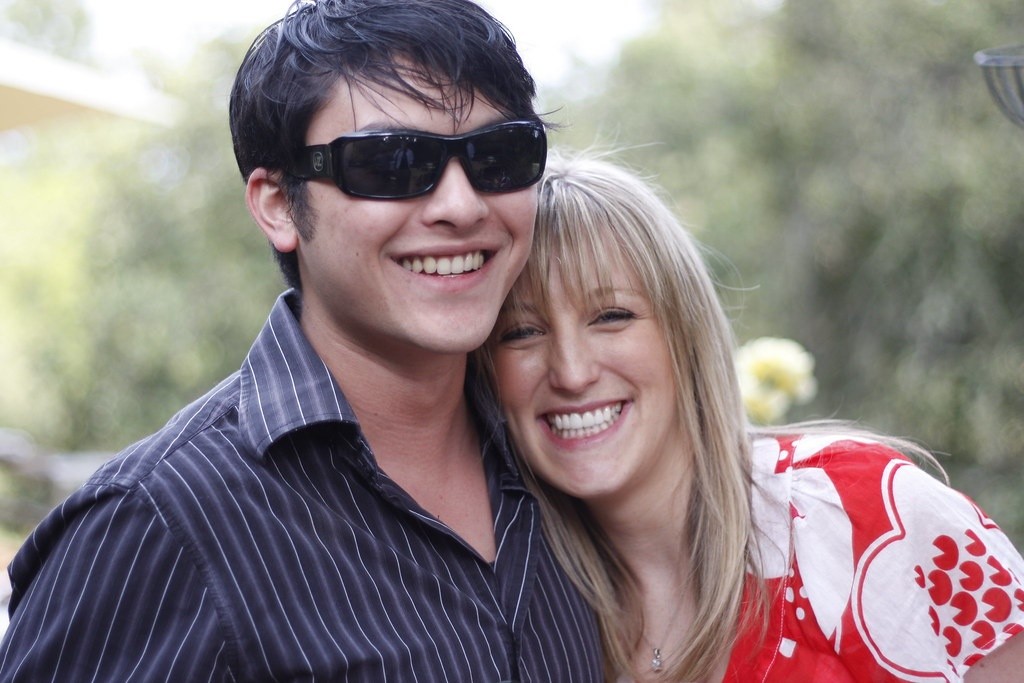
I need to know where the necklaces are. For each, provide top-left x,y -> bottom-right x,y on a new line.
642,576 -> 690,673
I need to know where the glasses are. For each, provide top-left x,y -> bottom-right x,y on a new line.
284,120 -> 548,201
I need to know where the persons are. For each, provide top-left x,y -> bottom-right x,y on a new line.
477,150 -> 1024,683
0,0 -> 613,683
